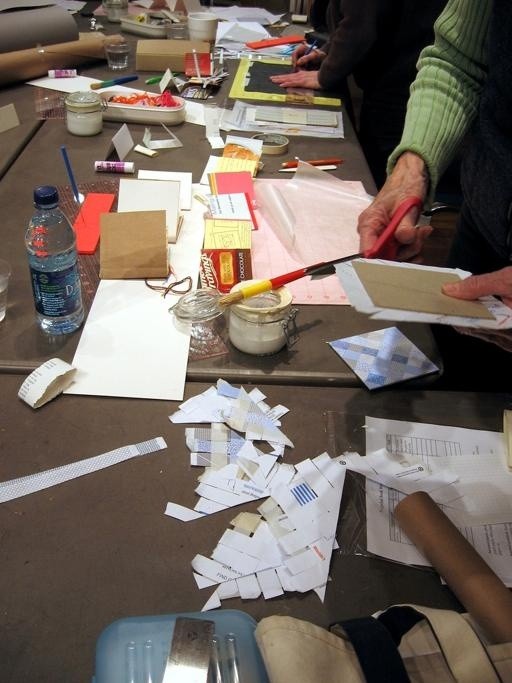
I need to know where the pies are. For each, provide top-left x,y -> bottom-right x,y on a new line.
91,74 -> 138,89
216,261 -> 326,307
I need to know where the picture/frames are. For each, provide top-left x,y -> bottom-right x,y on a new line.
1,0 -> 448,388
0,370 -> 511,682
0,1 -> 102,182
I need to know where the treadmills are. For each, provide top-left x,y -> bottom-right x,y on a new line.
163,613 -> 215,683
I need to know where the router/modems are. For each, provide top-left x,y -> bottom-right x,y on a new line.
250,134 -> 289,155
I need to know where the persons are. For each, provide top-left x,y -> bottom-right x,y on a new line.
353,2 -> 512,347
271,3 -> 444,187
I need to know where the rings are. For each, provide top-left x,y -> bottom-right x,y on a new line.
282,159 -> 344,169
304,39 -> 318,57
145,72 -> 180,84
207,633 -> 241,683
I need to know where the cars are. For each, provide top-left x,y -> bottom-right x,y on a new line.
187,12 -> 218,50
104,38 -> 130,69
0,260 -> 12,321
102,0 -> 128,21
167,23 -> 188,39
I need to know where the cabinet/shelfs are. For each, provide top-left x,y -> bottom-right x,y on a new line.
22,184 -> 85,337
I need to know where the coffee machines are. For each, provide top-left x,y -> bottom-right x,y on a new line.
306,197 -> 425,276
